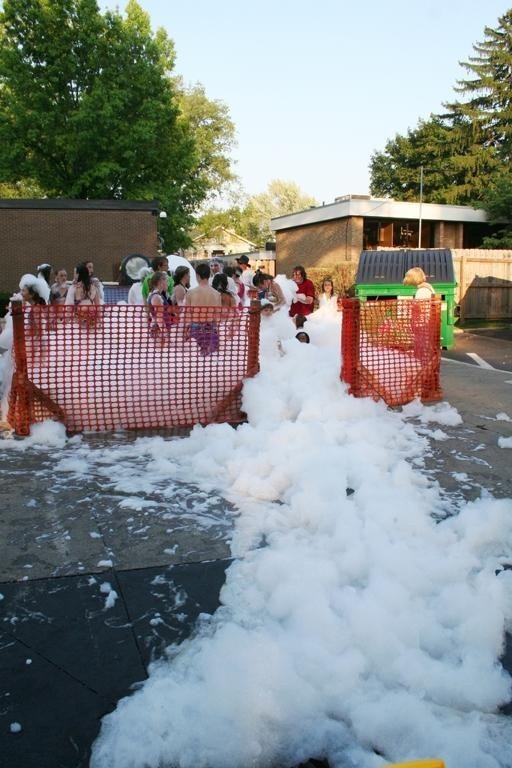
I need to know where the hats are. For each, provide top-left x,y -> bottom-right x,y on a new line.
234,253 -> 254,268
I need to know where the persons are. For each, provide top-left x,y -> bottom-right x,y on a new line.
403,264 -> 443,398
